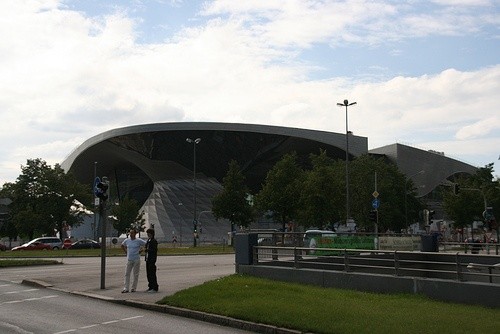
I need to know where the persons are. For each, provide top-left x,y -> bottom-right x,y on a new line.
0,220 -> 500,257
144,228 -> 159,293
121,228 -> 147,293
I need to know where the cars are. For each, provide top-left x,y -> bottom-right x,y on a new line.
11,237 -> 102,250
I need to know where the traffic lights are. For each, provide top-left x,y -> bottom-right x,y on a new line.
455,184 -> 461,195
95,181 -> 110,203
427,209 -> 435,226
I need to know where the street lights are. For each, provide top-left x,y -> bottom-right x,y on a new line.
186,137 -> 199,246
337,100 -> 357,228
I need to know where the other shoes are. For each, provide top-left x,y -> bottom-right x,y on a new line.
145,287 -> 159,292
131,287 -> 136,292
121,288 -> 129,293
